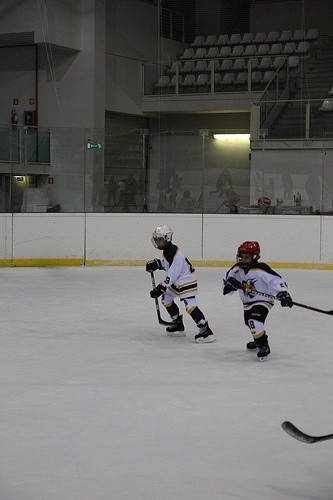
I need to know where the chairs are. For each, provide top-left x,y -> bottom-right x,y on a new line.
320,97 -> 333,111
154,27 -> 317,88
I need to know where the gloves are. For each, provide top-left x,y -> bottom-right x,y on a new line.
150,284 -> 167,298
222,277 -> 239,295
146,259 -> 162,272
276,291 -> 293,308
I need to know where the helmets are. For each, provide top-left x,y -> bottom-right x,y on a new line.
150,225 -> 173,249
236,241 -> 260,267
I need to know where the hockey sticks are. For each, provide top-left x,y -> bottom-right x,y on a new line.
236,285 -> 333,316
151,269 -> 175,326
281,421 -> 333,444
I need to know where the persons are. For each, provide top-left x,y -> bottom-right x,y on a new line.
155,164 -> 240,212
145,225 -> 216,343
222,240 -> 293,361
254,167 -> 321,215
107,166 -> 141,211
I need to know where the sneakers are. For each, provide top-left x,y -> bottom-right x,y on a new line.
245,331 -> 270,361
166,314 -> 186,337
194,320 -> 216,343
